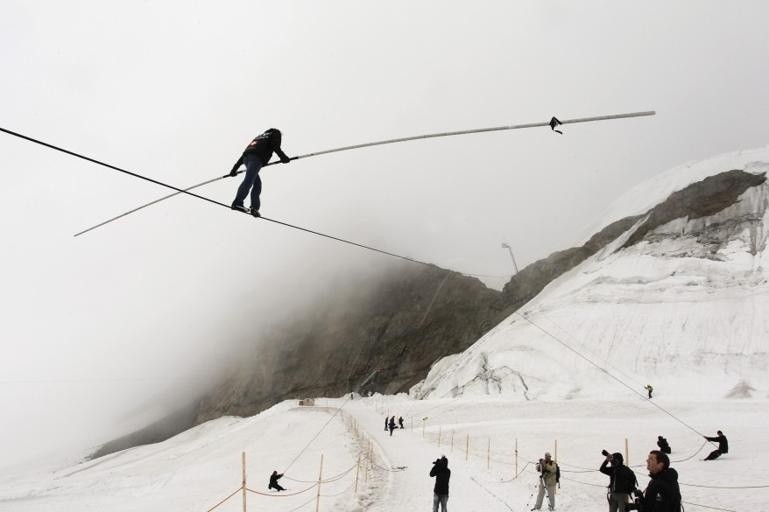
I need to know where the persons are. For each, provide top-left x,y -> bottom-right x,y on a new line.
704,430 -> 728,460
384,416 -> 404,436
599,452 -> 636,512
634,450 -> 681,512
268,471 -> 287,491
530,452 -> 557,511
430,456 -> 450,512
230,128 -> 290,216
657,436 -> 671,453
644,384 -> 653,398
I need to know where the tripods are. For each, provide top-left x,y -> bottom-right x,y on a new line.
523,473 -> 554,512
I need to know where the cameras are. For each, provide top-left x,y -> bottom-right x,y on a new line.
622,490 -> 647,512
601,449 -> 612,460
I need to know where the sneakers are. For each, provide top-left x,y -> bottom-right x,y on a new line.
231,203 -> 261,217
